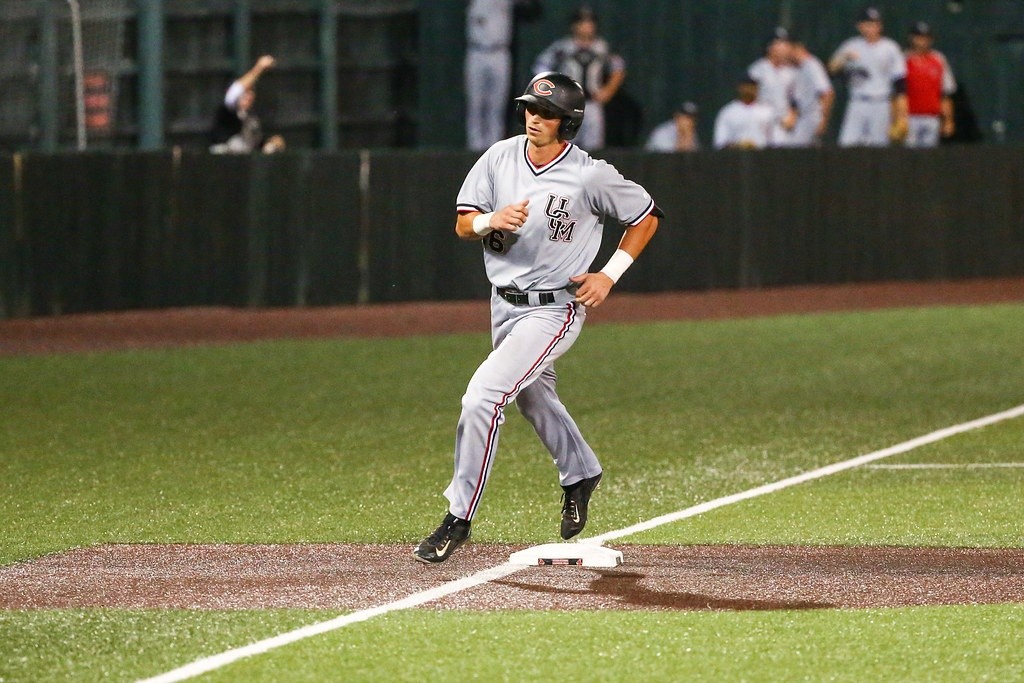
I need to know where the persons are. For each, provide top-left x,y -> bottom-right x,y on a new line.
537,9 -> 621,151
412,73 -> 665,565
212,56 -> 290,155
715,28 -> 833,144
827,7 -> 957,147
651,101 -> 699,152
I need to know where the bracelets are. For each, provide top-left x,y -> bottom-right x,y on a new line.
598,247 -> 632,285
473,212 -> 498,237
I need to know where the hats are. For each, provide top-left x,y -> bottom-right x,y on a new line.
910,22 -> 935,38
855,8 -> 881,23
767,28 -> 792,43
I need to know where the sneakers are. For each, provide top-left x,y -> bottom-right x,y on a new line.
560,471 -> 603,541
413,512 -> 471,562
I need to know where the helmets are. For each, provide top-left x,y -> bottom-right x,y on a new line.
516,71 -> 585,139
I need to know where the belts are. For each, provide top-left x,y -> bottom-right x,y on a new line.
497,287 -> 555,306
